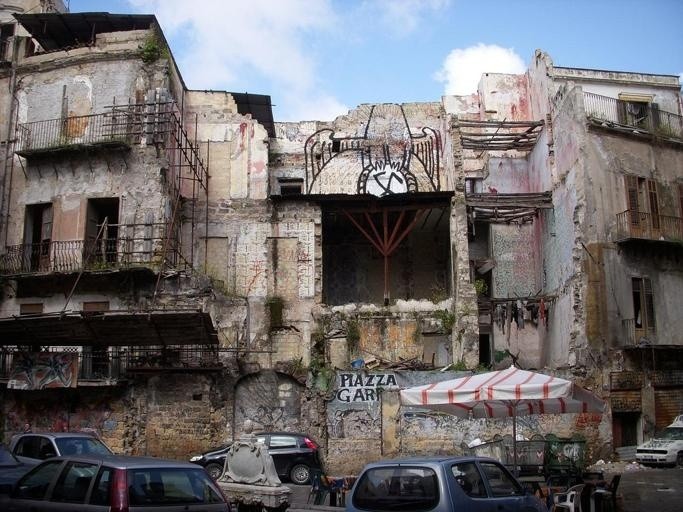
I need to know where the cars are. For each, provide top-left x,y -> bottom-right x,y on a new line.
0,432 -> 230,512
635,415 -> 683,467
345,457 -> 547,512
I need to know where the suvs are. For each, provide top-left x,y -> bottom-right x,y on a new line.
189,430 -> 322,486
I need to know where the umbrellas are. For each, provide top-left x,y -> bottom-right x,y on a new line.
398,365 -> 606,478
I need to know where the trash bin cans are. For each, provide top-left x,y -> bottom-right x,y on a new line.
468,433 -> 586,480
